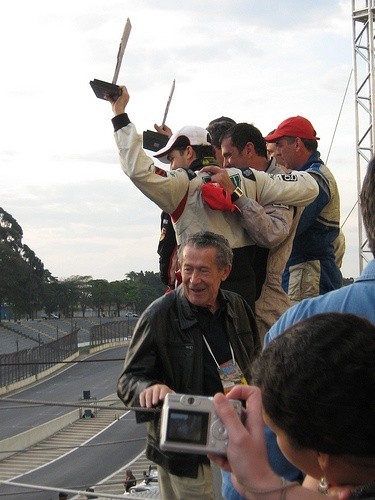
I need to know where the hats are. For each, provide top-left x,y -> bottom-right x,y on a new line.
152,125 -> 212,164
201,182 -> 242,216
265,115 -> 320,143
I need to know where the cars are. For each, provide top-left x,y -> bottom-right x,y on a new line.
42,314 -> 59,320
125,312 -> 138,318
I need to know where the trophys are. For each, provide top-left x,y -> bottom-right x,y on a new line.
89,17 -> 133,103
142,79 -> 177,152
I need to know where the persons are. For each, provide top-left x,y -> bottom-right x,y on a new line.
59,492 -> 68,500
85,488 -> 98,499
219,155 -> 375,500
212,383 -> 340,500
111,85 -> 345,346
123,470 -> 136,495
117,230 -> 264,500
250,312 -> 375,500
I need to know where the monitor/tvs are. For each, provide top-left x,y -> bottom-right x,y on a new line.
159,394 -> 243,458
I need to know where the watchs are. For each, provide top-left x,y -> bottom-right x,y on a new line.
230,186 -> 244,204
